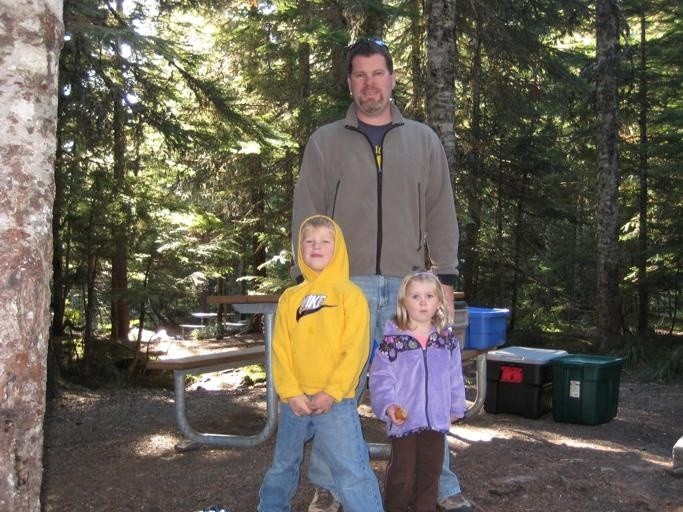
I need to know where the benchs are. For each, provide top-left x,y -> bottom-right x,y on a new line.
367,346 -> 495,463
149,345 -> 264,447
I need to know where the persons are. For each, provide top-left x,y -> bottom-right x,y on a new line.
369,270 -> 467,512
291,38 -> 469,512
258,216 -> 385,512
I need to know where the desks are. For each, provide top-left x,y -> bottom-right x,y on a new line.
207,293 -> 281,446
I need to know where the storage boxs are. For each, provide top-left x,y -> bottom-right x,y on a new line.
453,292 -> 468,350
551,354 -> 625,423
468,306 -> 508,350
485,345 -> 567,418
